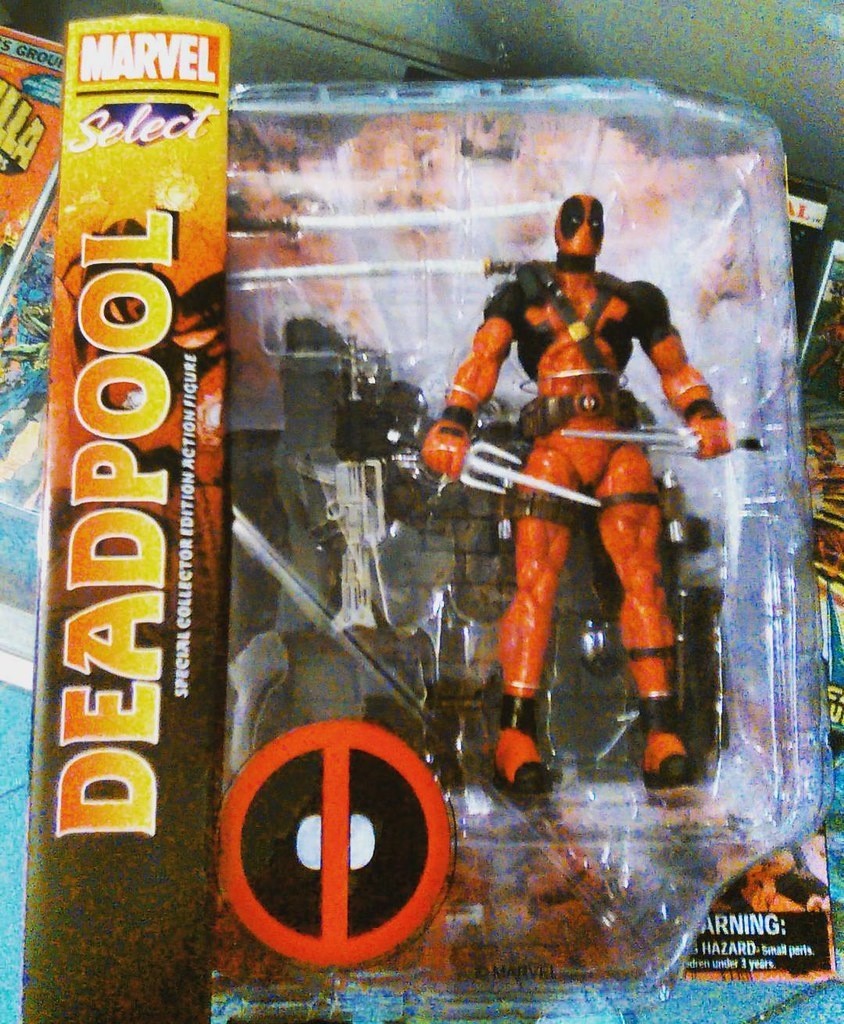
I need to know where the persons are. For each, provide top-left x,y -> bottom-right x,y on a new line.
423,197 -> 730,798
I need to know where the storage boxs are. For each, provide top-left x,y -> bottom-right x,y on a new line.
211,83 -> 829,991
